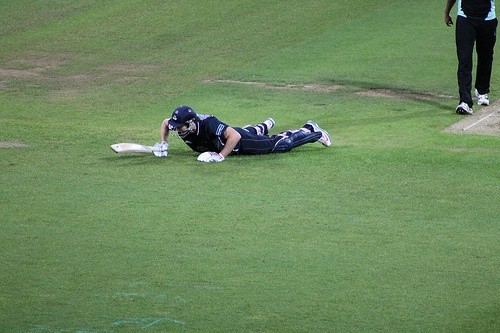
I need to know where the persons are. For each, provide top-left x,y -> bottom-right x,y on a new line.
152,105 -> 333,164
444,0 -> 499,117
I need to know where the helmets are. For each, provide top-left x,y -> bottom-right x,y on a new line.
168,106 -> 199,139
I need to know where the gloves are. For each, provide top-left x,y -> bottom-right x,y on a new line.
153,142 -> 168,157
196,151 -> 225,163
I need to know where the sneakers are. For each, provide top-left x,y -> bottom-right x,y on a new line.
305,120 -> 332,147
474,89 -> 489,106
456,101 -> 473,115
264,118 -> 275,135
